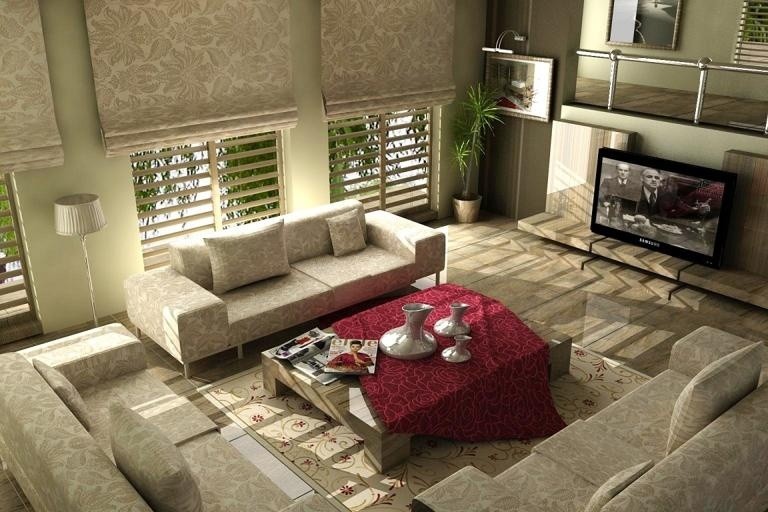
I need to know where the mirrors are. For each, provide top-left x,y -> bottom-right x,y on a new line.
483,53 -> 555,123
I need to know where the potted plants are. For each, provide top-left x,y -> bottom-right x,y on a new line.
448,83 -> 504,224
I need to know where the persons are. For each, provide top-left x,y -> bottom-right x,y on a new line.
601,162 -> 642,203
634,168 -> 713,217
328,340 -> 376,374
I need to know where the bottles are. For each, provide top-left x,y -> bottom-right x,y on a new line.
440,334 -> 474,363
432,302 -> 472,338
378,302 -> 438,361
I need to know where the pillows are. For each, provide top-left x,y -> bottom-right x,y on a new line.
582,461 -> 651,510
326,209 -> 367,257
202,218 -> 291,294
111,400 -> 202,510
31,358 -> 92,427
661,337 -> 761,456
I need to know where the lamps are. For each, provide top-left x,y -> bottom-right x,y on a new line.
54,193 -> 110,325
482,29 -> 525,55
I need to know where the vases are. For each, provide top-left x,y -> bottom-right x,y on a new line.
381,301 -> 472,362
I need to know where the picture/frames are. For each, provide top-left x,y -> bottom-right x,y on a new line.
605,1 -> 683,51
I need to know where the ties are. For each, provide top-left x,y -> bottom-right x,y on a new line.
648,190 -> 656,206
620,178 -> 625,189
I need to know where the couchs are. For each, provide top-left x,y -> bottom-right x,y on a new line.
123,199 -> 445,379
408,327 -> 768,512
1,319 -> 335,509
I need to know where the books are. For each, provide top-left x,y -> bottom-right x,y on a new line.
271,326 -> 354,386
324,337 -> 379,373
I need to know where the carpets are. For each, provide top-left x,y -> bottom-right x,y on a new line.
197,338 -> 654,508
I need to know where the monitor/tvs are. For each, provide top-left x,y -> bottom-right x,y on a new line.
590,146 -> 739,271
